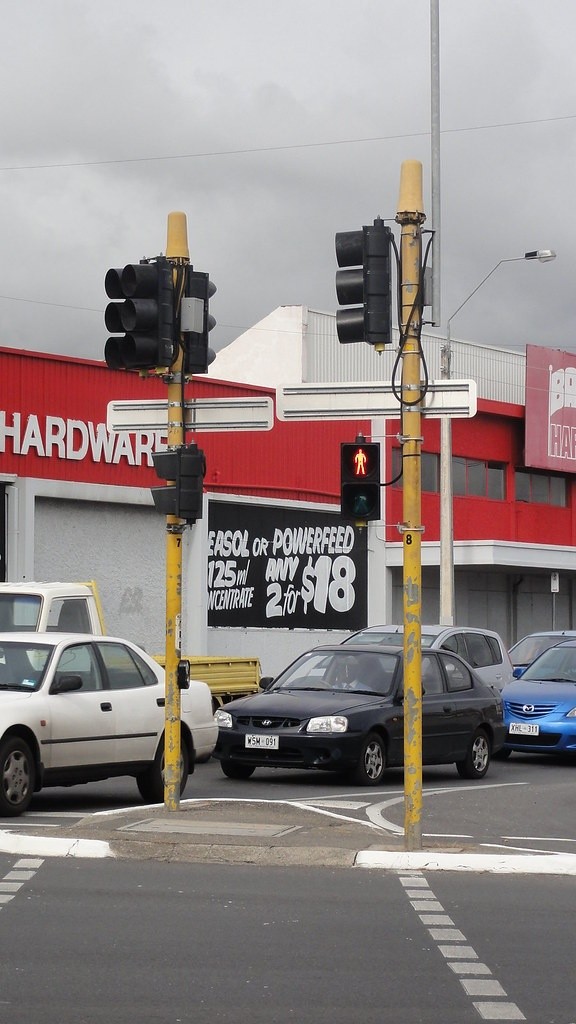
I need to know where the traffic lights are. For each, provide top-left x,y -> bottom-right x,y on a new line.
338,442 -> 383,525
95,263 -> 170,370
329,225 -> 393,347
147,446 -> 208,519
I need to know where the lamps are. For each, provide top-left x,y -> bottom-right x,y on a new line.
446,248 -> 557,359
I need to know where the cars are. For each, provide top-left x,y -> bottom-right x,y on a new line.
0,620 -> 576,820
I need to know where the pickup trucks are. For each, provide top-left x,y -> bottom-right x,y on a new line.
0,579 -> 263,711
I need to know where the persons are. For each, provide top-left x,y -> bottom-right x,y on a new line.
335,656 -> 371,691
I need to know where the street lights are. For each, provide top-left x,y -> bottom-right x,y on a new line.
439,245 -> 559,630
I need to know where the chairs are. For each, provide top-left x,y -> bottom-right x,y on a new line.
357,656 -> 386,690
